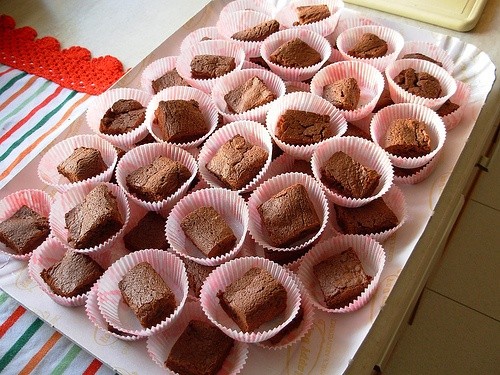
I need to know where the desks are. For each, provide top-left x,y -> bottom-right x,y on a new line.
1,1 -> 500,375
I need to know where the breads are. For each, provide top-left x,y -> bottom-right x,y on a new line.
0,1 -> 467,375
